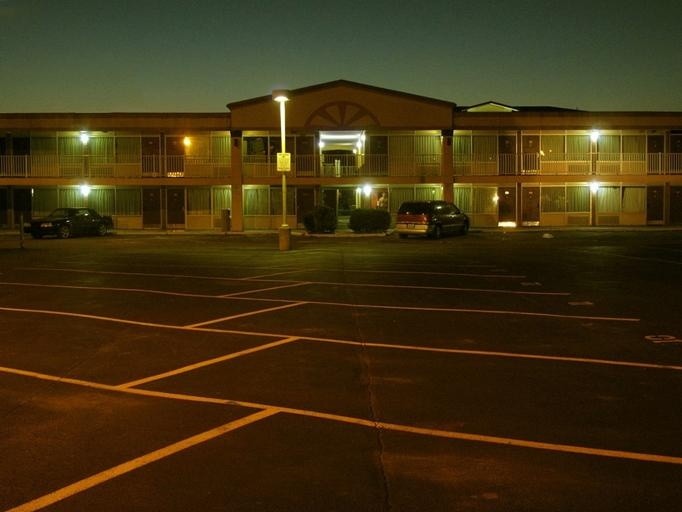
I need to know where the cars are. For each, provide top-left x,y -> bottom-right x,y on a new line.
23,207 -> 114,238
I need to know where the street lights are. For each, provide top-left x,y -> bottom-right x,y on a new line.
270,87 -> 293,253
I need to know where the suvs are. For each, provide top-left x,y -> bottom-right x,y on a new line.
395,199 -> 470,239
